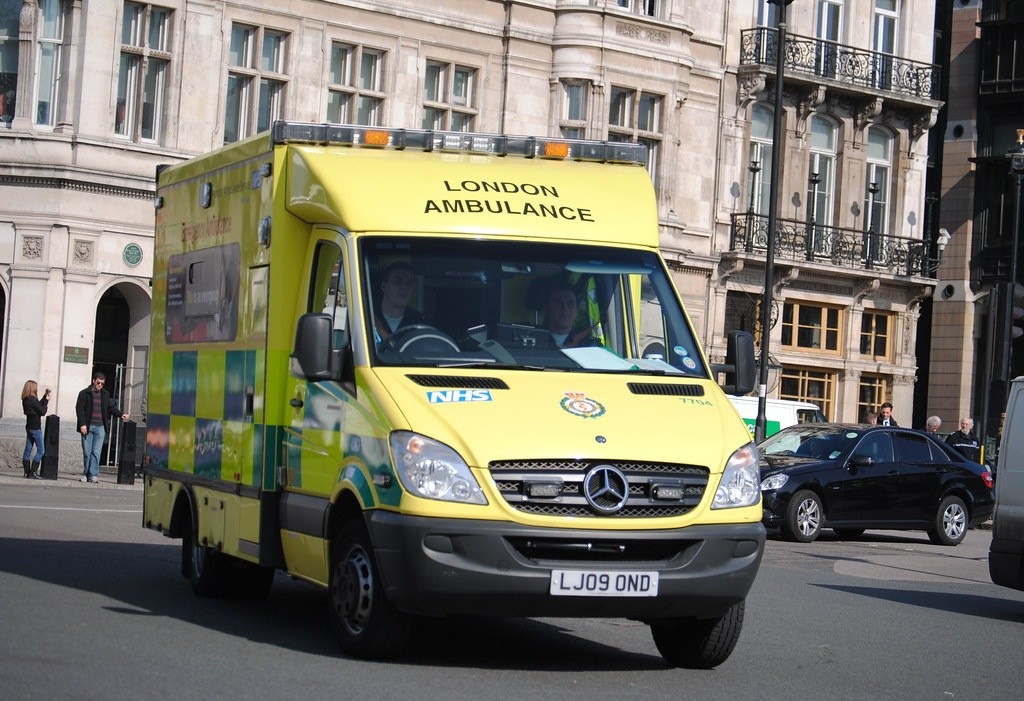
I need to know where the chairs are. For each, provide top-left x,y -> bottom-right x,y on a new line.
859,442 -> 879,461
525,277 -> 551,324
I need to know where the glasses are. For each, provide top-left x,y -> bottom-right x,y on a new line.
95,380 -> 104,385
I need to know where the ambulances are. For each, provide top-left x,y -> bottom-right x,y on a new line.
139,119 -> 769,671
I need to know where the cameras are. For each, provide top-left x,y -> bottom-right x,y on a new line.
46,389 -> 51,396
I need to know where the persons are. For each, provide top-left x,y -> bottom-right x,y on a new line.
510,276 -> 605,365
864,401 -> 900,427
373,262 -> 438,354
808,438 -> 825,459
921,414 -> 941,443
76,373 -> 130,484
945,417 -> 981,462
21,380 -> 51,480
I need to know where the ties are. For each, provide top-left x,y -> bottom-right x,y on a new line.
885,421 -> 888,425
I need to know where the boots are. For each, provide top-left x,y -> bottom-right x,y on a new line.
28,461 -> 42,479
22,460 -> 31,477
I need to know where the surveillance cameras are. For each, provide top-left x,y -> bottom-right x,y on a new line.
939,227 -> 951,239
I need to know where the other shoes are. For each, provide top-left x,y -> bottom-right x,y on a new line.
89,475 -> 99,482
79,475 -> 87,482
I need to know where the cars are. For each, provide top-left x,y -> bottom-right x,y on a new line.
755,421 -> 996,544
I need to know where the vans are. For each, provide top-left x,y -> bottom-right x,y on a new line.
987,376 -> 1024,592
725,393 -> 830,455
